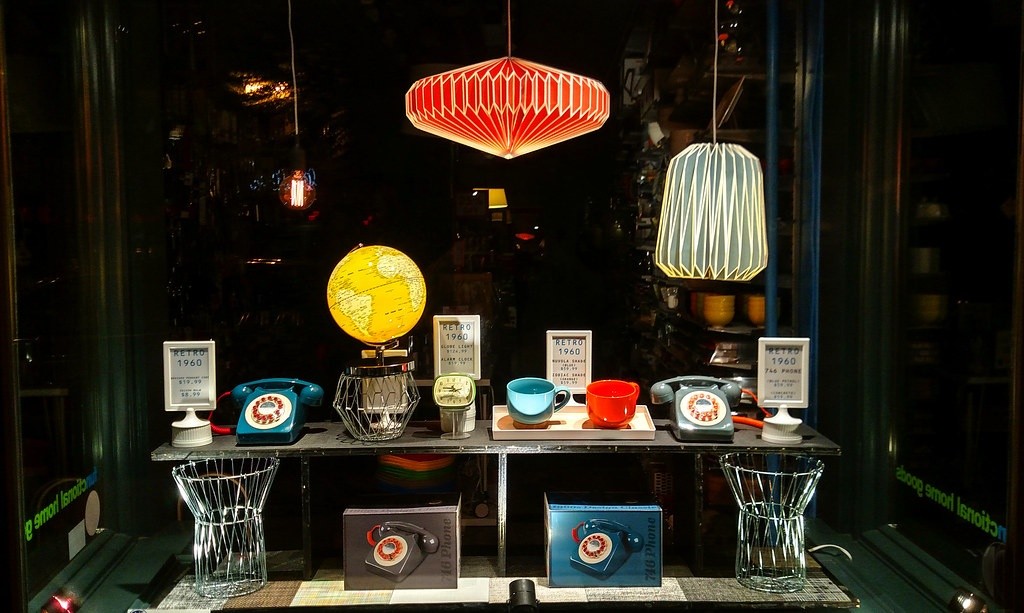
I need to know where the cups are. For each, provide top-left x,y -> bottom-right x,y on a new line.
691,293 -> 734,329
745,295 -> 765,329
586,380 -> 641,429
506,377 -> 571,425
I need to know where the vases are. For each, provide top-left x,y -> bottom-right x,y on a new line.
331,367 -> 423,441
719,452 -> 826,591
171,457 -> 281,599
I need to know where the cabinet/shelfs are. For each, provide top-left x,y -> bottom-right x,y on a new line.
151,417 -> 864,613
614,1 -> 861,464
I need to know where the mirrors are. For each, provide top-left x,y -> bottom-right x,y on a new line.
848,0 -> 1024,613
0,0 -> 141,613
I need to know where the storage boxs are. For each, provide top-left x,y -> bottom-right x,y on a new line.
544,491 -> 665,588
343,491 -> 461,591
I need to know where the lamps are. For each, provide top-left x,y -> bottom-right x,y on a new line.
327,244 -> 425,428
404,0 -> 611,161
655,0 -> 769,281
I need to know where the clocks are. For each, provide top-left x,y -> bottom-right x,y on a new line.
433,372 -> 476,407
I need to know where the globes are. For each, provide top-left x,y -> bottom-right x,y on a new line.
325,244 -> 427,379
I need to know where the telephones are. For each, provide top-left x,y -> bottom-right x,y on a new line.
230,377 -> 325,448
650,374 -> 742,442
